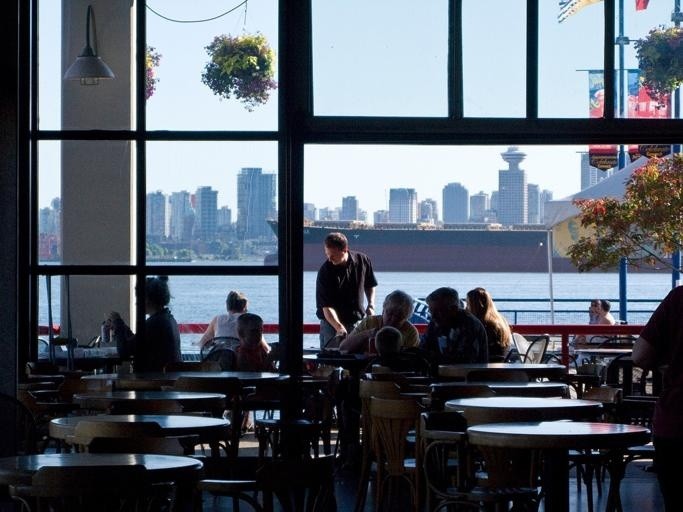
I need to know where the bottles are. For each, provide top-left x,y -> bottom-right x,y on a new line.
100,312 -> 111,343
368,330 -> 375,355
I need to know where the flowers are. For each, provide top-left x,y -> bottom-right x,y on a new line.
634,24 -> 682,112
143,43 -> 163,100
199,30 -> 276,114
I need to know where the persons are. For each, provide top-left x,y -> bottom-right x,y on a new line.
569,300 -> 617,368
338,289 -> 417,349
312,229 -> 380,351
418,285 -> 489,378
231,309 -> 276,370
630,283 -> 682,511
196,288 -> 269,435
362,326 -> 419,371
551,298 -> 601,364
461,287 -> 512,350
102,276 -> 183,377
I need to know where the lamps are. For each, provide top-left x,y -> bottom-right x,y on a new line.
63,4 -> 117,89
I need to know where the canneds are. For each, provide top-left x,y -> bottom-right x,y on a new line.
101,324 -> 110,344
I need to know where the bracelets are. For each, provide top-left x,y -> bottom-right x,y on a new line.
367,303 -> 375,311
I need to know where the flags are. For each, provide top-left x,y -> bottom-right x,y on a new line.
557,0 -> 603,23
